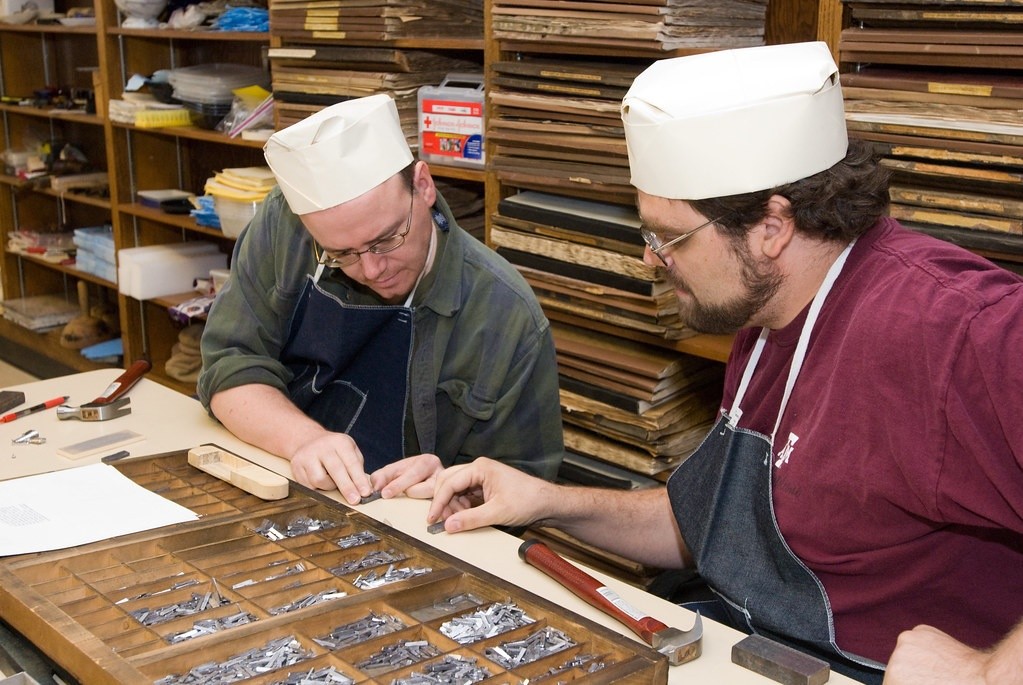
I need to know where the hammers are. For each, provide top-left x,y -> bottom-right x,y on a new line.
57,357 -> 152,421
518,538 -> 703,667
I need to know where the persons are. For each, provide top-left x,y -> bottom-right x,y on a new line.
195,95 -> 566,507
427,40 -> 1023,685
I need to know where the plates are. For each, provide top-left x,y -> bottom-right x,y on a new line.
60,16 -> 97,27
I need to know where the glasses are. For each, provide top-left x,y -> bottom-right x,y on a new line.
640,192 -> 791,268
313,181 -> 417,269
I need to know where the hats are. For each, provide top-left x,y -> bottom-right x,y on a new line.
261,94 -> 415,215
620,40 -> 849,200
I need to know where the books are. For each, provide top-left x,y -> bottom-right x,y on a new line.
269,0 -> 1022,587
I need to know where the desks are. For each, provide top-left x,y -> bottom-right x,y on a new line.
0,369 -> 865,684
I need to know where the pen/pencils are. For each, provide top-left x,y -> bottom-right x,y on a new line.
0,395 -> 70,425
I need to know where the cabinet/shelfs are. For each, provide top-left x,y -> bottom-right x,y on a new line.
0,0 -> 851,582
